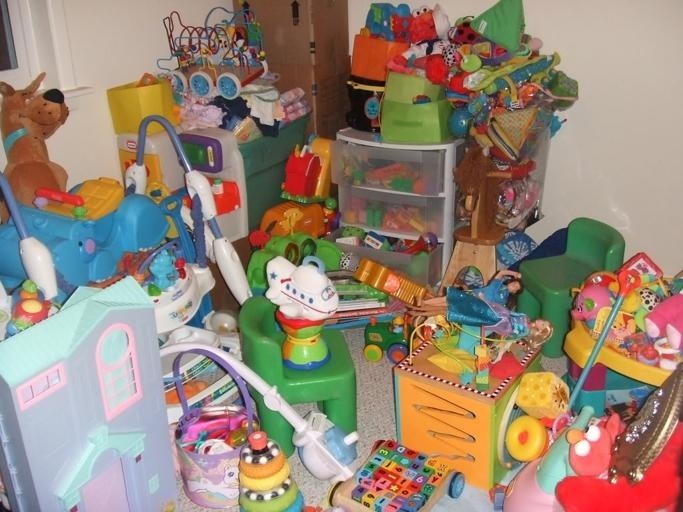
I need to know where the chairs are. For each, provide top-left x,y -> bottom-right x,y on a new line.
236,294 -> 360,456
517,217 -> 628,361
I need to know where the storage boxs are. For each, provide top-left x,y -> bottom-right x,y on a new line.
235,117 -> 310,234
105,76 -> 176,136
173,407 -> 264,508
377,99 -> 451,144
331,130 -> 471,289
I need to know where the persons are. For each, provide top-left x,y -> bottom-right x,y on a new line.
404,286 -> 553,365
424,271 -> 523,306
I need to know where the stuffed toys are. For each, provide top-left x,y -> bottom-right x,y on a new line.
554,413 -> 683,512
1,71 -> 69,225
643,291 -> 683,350
394,1 -> 541,99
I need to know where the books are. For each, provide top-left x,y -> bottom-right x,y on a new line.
324,280 -> 406,325
